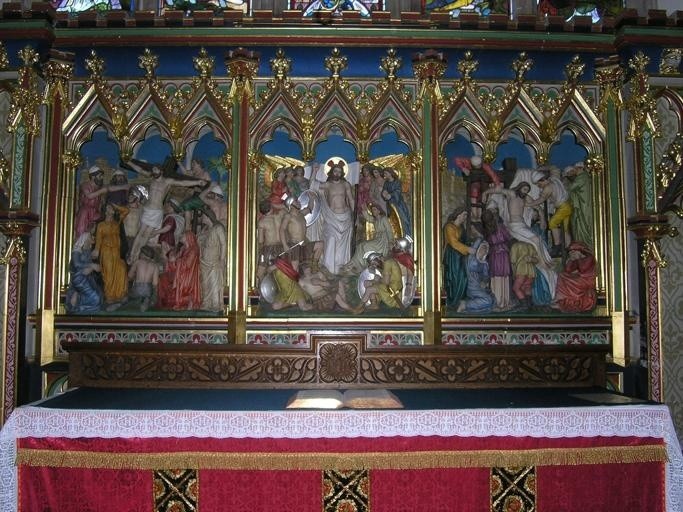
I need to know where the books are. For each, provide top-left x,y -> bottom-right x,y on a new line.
284,388 -> 404,409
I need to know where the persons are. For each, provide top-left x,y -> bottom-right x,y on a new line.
62,161 -> 229,312
438,156 -> 599,313
257,160 -> 413,315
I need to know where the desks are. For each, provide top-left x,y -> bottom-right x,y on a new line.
2,388 -> 680,511
